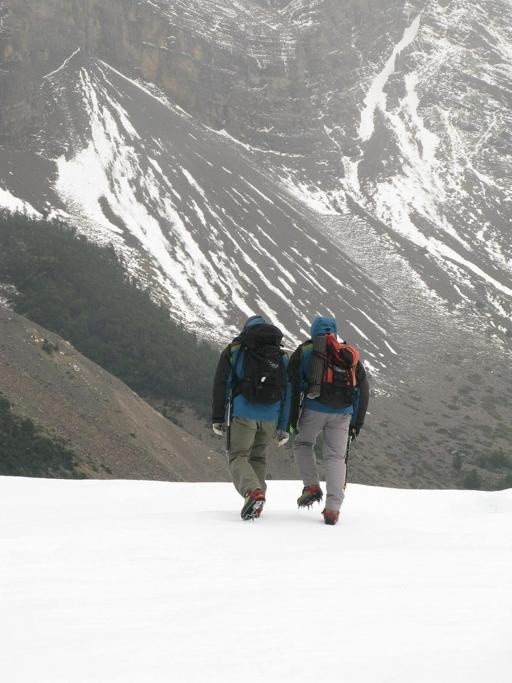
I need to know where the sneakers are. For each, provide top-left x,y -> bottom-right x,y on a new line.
297,486 -> 323,506
240,490 -> 266,520
321,508 -> 340,525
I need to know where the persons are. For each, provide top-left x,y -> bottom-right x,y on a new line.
212,315 -> 293,520
286,316 -> 369,525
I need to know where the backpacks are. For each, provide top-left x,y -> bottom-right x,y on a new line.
307,336 -> 359,408
239,323 -> 284,403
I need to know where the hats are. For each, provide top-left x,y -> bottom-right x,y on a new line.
243,315 -> 265,330
310,316 -> 338,339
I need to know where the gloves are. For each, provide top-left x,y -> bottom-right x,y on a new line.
349,428 -> 357,442
212,423 -> 223,436
273,430 -> 289,447
288,423 -> 298,434
